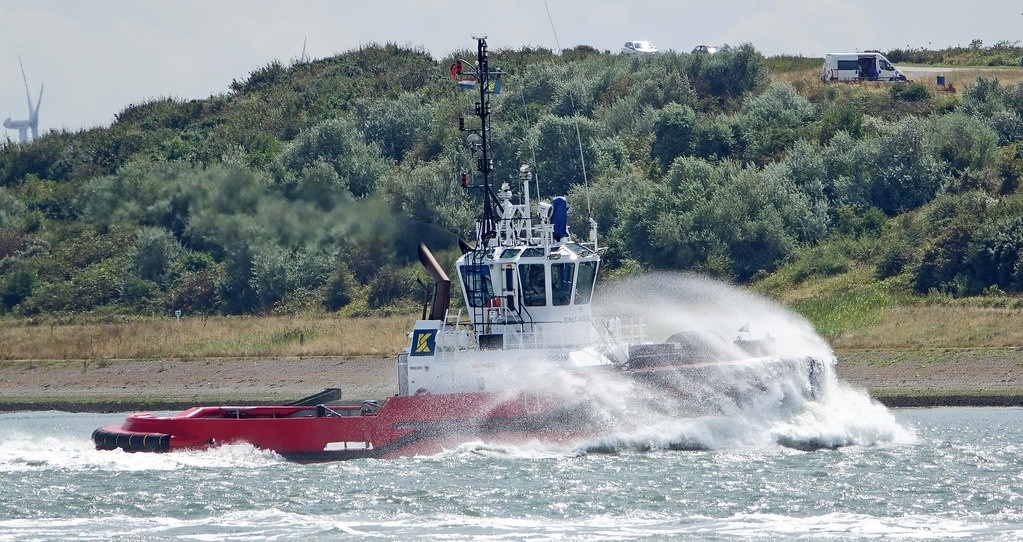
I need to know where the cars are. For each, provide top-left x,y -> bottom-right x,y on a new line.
619,41 -> 659,55
691,44 -> 719,54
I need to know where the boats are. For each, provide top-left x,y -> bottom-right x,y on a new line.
92,0 -> 826,464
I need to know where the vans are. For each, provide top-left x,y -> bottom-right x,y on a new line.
821,53 -> 906,81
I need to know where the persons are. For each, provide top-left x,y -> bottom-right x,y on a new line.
859,62 -> 879,81
